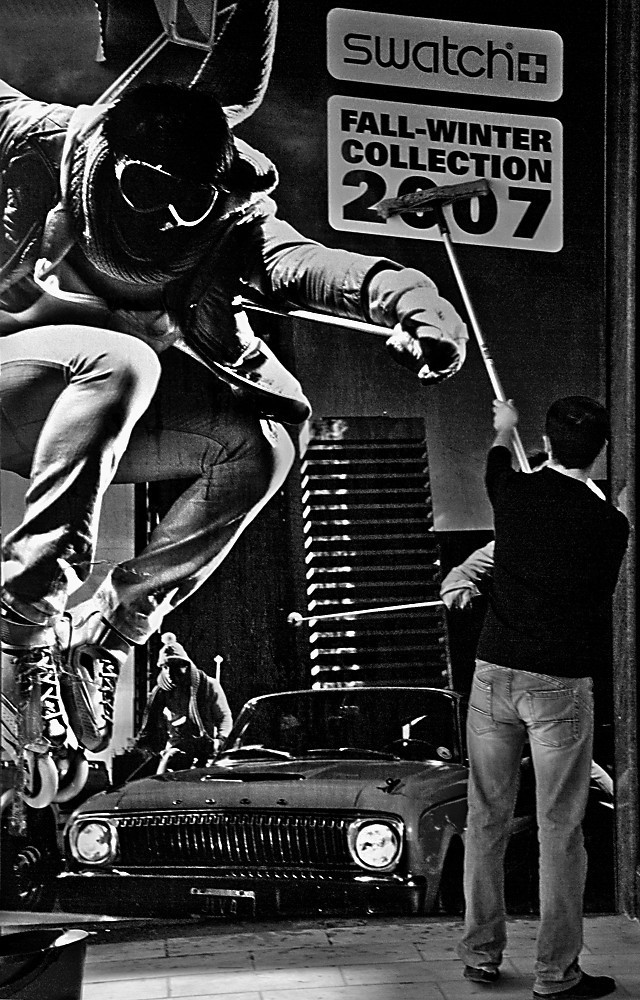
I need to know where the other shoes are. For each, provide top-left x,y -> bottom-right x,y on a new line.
533,971 -> 617,1000
463,964 -> 502,984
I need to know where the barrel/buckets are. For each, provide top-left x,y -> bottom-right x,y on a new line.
0,928 -> 90,1000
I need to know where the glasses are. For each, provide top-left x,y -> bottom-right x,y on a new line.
112,153 -> 228,230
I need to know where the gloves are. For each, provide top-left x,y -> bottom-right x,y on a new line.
369,267 -> 470,386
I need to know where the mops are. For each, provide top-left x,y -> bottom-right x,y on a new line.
377,177 -> 531,473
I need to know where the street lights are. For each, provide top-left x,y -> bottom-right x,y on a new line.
284,599 -> 460,758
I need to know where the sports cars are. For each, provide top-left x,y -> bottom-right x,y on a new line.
53,681 -> 538,922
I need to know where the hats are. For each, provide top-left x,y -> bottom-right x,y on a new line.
155,631 -> 190,667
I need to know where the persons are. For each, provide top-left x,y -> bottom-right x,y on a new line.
125,632 -> 234,773
454,395 -> 629,1000
0,76 -> 470,808
440,450 -> 619,921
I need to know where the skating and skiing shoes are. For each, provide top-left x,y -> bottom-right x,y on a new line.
53,596 -> 131,804
0,607 -> 68,806
